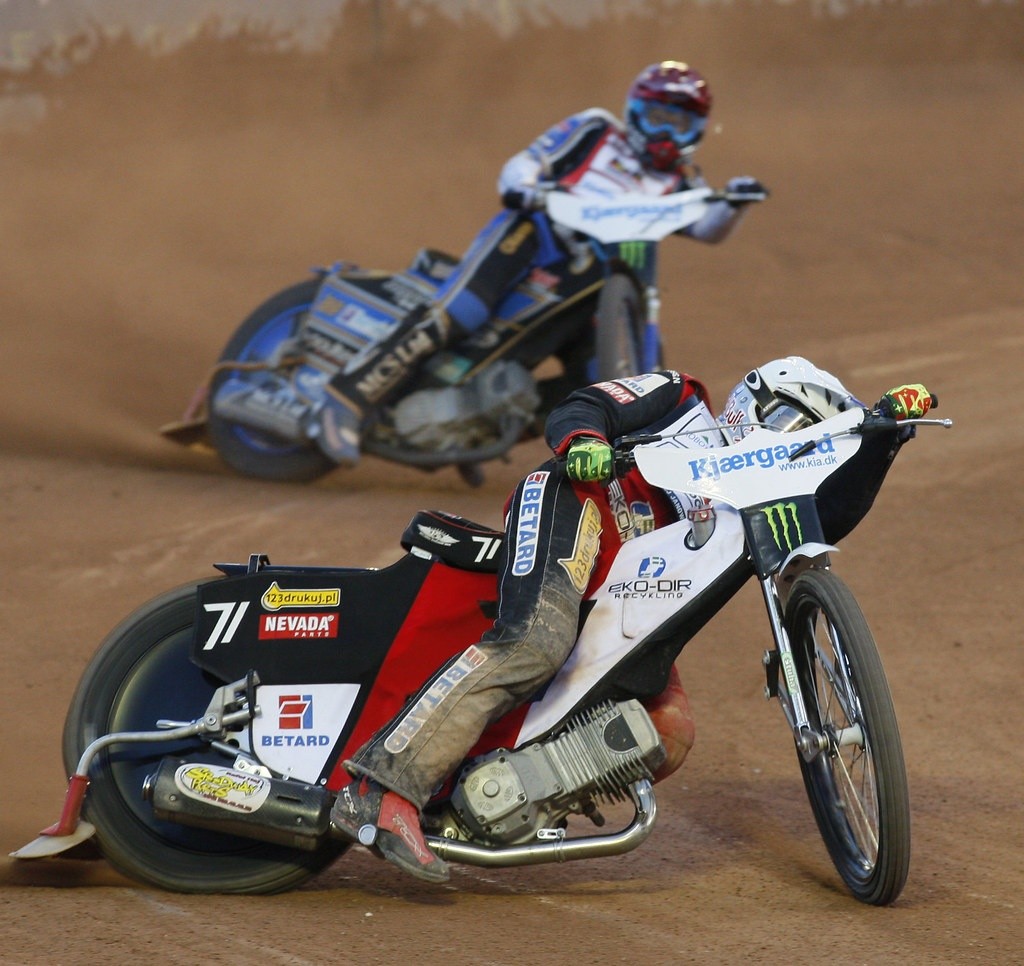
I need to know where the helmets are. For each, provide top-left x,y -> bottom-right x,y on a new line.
625,60 -> 713,172
715,356 -> 868,448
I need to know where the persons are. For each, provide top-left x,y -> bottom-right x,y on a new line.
331,354 -> 932,884
299,60 -> 770,470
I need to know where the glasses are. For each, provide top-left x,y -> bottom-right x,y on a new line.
759,398 -> 814,433
637,99 -> 699,143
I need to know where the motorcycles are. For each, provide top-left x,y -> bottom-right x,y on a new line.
6,395 -> 953,906
156,183 -> 769,493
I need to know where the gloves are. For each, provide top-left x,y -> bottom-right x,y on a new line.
513,182 -> 557,209
566,435 -> 611,483
874,383 -> 932,421
725,175 -> 769,210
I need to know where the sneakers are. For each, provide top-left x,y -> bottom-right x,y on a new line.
303,387 -> 362,466
329,773 -> 450,883
